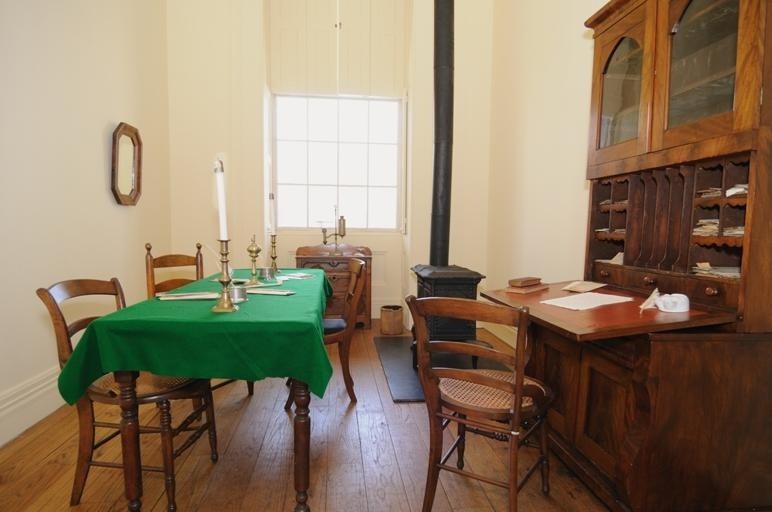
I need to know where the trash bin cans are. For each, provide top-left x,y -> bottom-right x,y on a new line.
380,305 -> 403,335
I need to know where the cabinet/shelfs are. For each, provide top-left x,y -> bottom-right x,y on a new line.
584,0 -> 772,166
293,246 -> 372,329
583,129 -> 772,334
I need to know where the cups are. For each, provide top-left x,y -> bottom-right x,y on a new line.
229,287 -> 247,304
259,267 -> 274,280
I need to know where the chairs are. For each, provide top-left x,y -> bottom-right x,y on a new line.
36,277 -> 217,512
405,296 -> 549,512
283,258 -> 367,409
145,242 -> 253,421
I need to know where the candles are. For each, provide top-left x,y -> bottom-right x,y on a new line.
214,159 -> 228,241
269,193 -> 276,236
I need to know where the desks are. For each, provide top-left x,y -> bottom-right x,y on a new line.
57,268 -> 334,512
480,278 -> 772,512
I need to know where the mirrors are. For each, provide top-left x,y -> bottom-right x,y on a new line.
110,122 -> 144,205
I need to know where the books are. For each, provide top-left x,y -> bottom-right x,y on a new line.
505,277 -> 550,295
